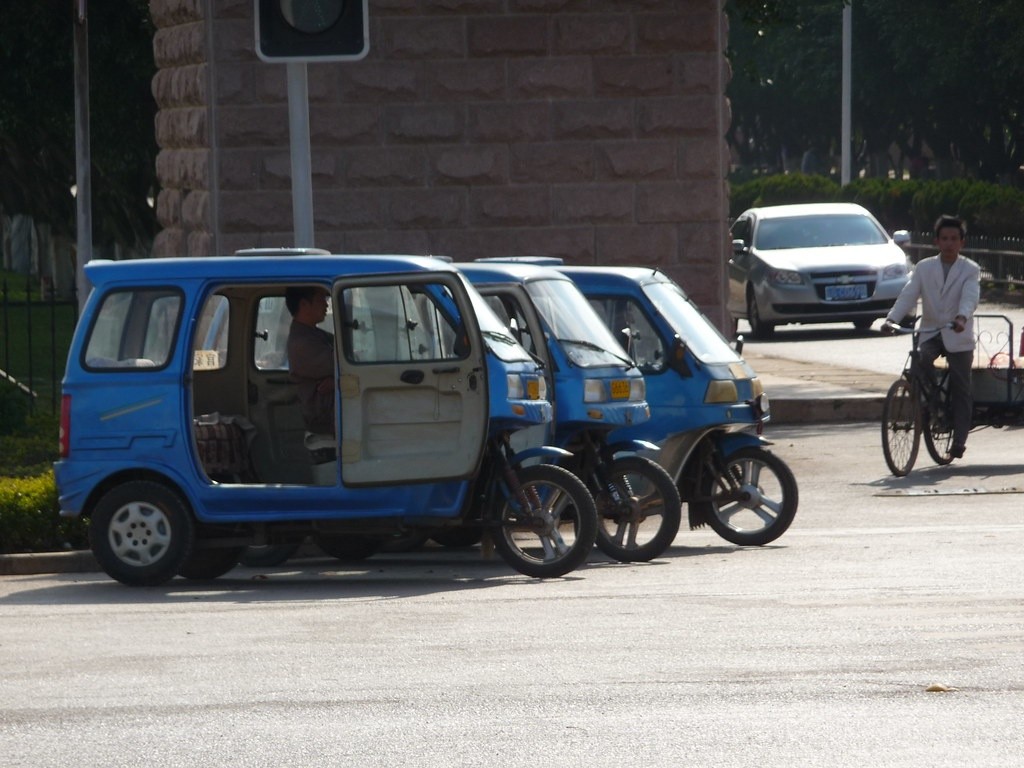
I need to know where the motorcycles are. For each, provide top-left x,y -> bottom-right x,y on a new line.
52,253 -> 601,589
472,255 -> 800,548
201,248 -> 682,562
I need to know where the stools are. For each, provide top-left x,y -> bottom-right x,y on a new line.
304,430 -> 337,484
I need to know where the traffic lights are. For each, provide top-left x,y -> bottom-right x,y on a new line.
252,1 -> 372,67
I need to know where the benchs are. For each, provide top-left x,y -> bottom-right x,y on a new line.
87,358 -> 247,479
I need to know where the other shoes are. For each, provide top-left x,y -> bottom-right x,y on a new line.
949,439 -> 966,458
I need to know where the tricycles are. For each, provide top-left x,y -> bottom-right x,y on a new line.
881,315 -> 1024,477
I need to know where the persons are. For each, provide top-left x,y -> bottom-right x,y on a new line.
881,215 -> 981,458
286,286 -> 340,435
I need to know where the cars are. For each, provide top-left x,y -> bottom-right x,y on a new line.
725,202 -> 918,341
